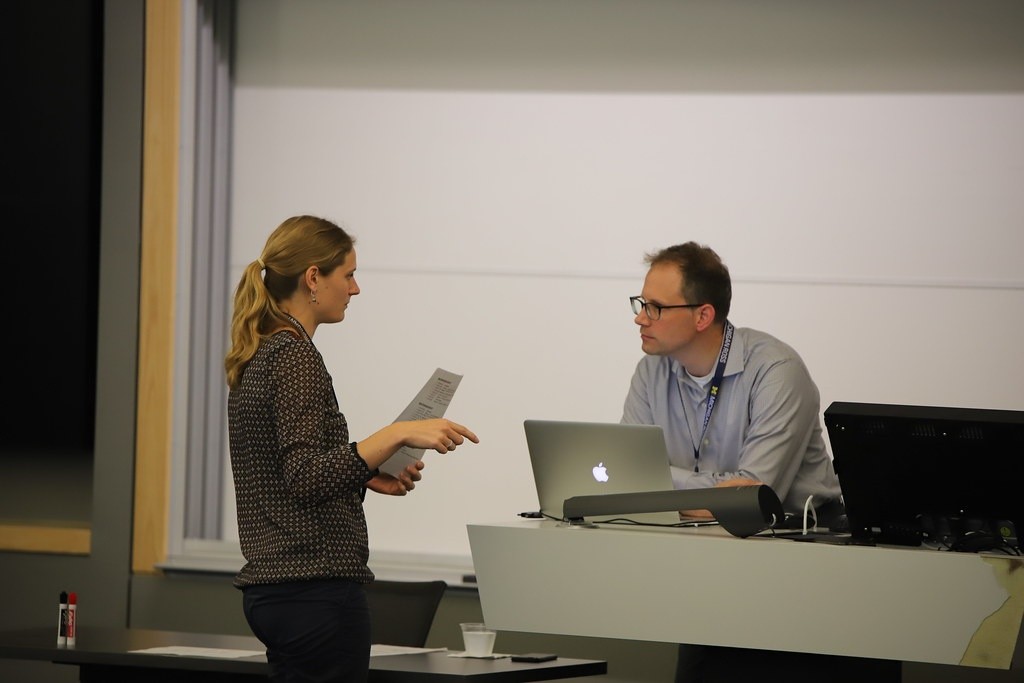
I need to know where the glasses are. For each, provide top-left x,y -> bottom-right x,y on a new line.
629,295 -> 705,319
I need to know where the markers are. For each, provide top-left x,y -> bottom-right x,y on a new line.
67,591 -> 79,649
57,591 -> 66,647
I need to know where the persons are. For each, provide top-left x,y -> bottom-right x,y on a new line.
226,215 -> 479,679
620,242 -> 847,524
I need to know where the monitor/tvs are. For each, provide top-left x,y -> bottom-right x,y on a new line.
825,401 -> 1024,549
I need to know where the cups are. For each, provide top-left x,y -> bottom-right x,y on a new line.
459,622 -> 498,656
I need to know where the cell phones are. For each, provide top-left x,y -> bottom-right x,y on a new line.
512,652 -> 558,663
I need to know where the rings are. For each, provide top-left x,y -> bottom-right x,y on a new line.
447,440 -> 452,447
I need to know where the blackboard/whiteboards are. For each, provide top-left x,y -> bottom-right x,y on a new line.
161,0 -> 1022,586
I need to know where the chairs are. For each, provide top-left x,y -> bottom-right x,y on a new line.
364,579 -> 448,648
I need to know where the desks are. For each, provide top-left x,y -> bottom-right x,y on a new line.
1,627 -> 610,681
467,518 -> 1023,683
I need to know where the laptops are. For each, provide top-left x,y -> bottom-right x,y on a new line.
524,419 -> 719,526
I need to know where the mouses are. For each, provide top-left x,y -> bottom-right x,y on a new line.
829,514 -> 849,532
